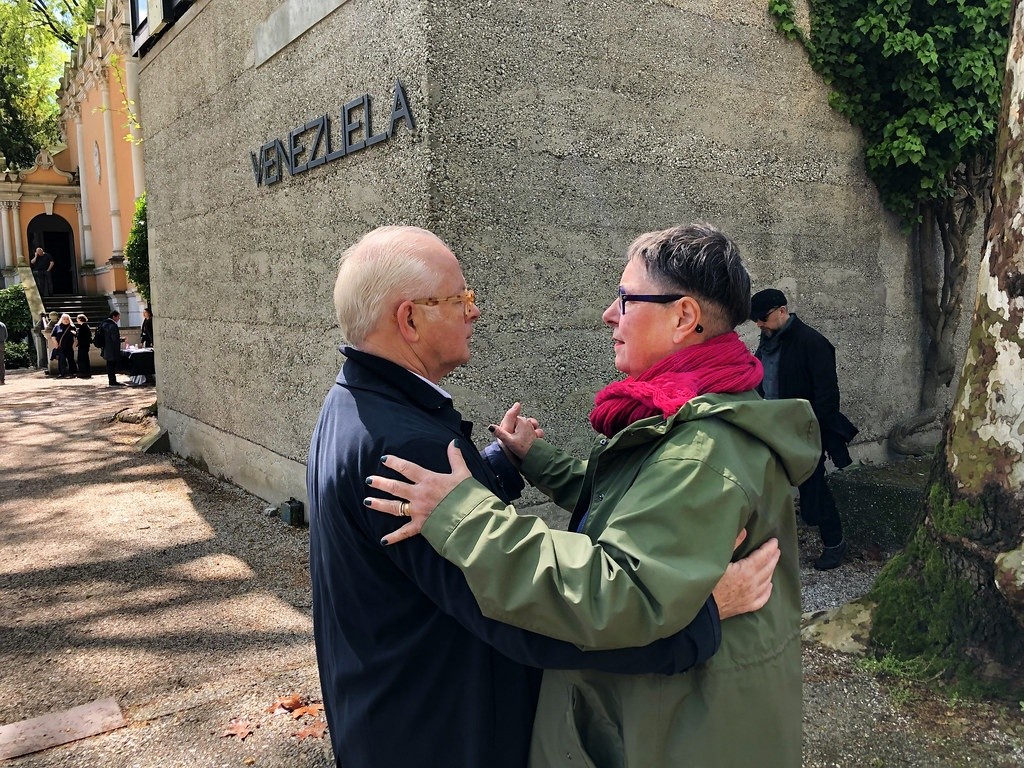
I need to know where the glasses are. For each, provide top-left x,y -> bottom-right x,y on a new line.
751,305 -> 782,323
617,286 -> 703,332
392,290 -> 475,324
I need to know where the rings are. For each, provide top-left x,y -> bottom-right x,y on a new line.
398,502 -> 410,517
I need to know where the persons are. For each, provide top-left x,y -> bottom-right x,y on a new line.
0,321 -> 8,386
98,311 -> 126,387
358,223 -> 823,768
75,314 -> 93,380
747,288 -> 859,572
30,247 -> 55,296
139,308 -> 155,349
303,226 -> 786,768
49,313 -> 78,379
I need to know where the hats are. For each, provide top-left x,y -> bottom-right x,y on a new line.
747,288 -> 787,316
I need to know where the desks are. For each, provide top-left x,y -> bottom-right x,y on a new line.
118,347 -> 156,387
